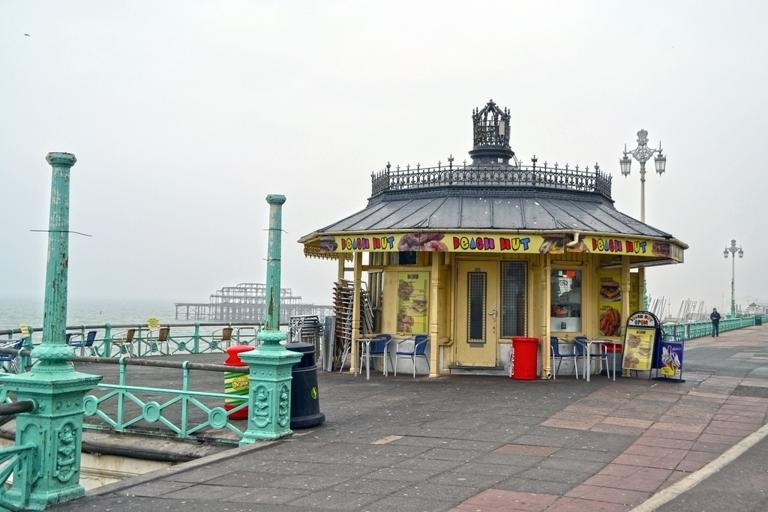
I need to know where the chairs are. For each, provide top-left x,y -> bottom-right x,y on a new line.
394,335 -> 430,380
67,330 -> 98,358
210,328 -> 233,354
331,277 -> 394,378
111,327 -> 173,359
0,338 -> 26,373
538,334 -> 616,382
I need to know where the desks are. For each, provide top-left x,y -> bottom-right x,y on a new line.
355,338 -> 381,380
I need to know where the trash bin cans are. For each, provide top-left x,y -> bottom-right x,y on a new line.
285,341 -> 325,430
511,337 -> 539,380
223,345 -> 256,420
755,316 -> 761,326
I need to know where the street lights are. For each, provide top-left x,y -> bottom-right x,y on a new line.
723,239 -> 744,317
620,127 -> 667,311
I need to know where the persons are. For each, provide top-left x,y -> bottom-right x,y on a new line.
709,308 -> 720,337
